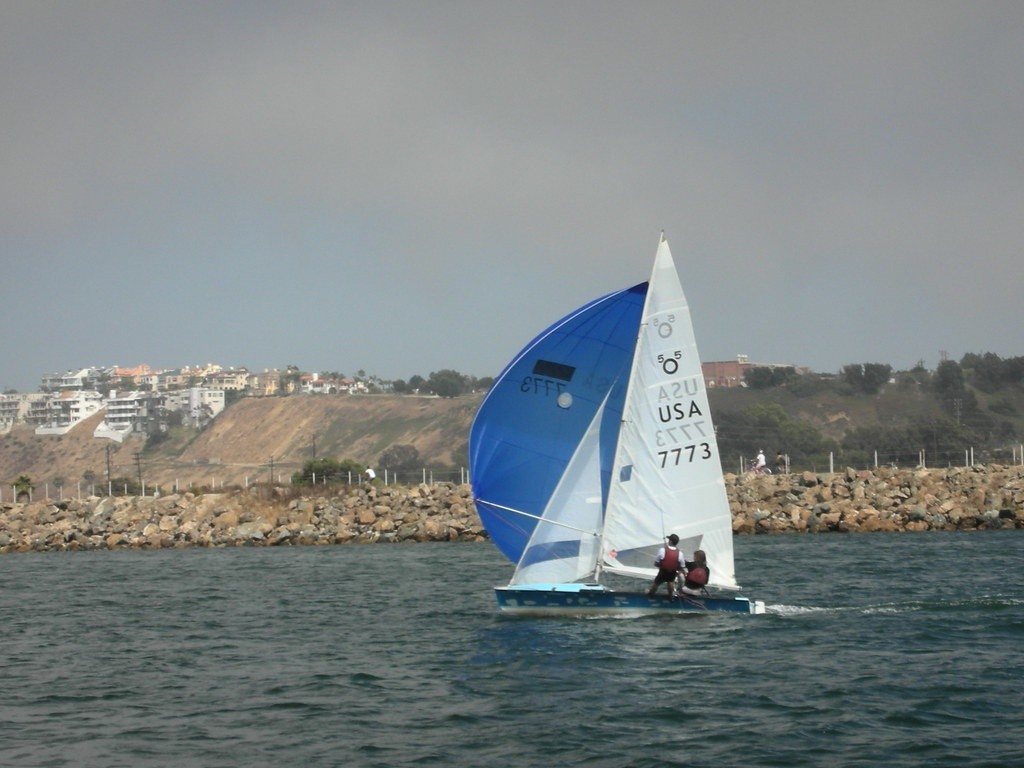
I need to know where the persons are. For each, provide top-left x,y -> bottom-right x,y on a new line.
364,466 -> 375,483
681,551 -> 710,596
648,534 -> 685,603
753,449 -> 786,474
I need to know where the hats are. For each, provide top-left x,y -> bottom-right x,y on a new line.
666,534 -> 679,544
760,450 -> 763,454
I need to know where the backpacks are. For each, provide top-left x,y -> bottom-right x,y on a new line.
688,561 -> 708,586
659,542 -> 680,573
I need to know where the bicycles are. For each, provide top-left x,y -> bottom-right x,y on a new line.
749,460 -> 772,475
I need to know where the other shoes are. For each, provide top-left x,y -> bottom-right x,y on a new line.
664,592 -> 674,603
644,588 -> 655,599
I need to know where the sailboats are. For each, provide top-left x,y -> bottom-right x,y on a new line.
465,228 -> 781,619
